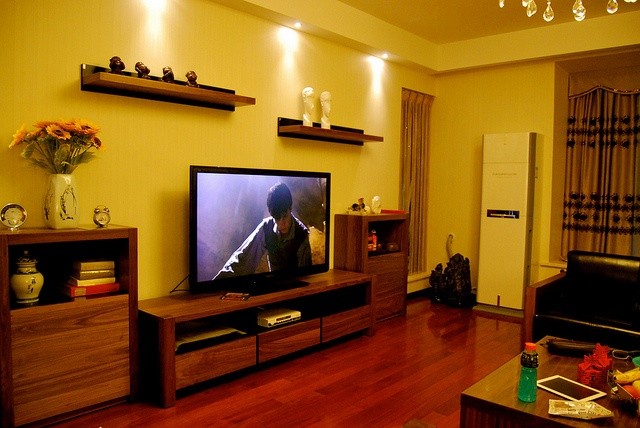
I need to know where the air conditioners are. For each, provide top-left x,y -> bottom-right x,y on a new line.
476,132 -> 537,311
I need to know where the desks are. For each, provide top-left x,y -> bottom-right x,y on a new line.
459,337 -> 640,428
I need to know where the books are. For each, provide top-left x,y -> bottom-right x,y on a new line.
64,282 -> 120,298
73,261 -> 115,271
67,276 -> 116,287
72,270 -> 115,280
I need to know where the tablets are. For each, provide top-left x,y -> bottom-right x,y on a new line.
536,374 -> 607,402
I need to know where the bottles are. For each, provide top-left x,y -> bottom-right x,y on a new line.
517,342 -> 538,403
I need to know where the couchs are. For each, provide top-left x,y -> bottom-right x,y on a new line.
523,249 -> 640,350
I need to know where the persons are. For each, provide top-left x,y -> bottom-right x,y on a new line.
211,182 -> 313,281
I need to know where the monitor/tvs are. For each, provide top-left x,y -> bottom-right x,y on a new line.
189,165 -> 331,296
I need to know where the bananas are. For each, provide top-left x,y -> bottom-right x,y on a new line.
613,367 -> 640,385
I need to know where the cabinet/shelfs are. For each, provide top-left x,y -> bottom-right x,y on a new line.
334,208 -> 410,324
137,270 -> 378,408
80,63 -> 385,145
2,222 -> 138,427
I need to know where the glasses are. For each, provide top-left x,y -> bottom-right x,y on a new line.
612,349 -> 640,360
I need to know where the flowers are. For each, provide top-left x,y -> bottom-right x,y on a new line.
9,117 -> 105,173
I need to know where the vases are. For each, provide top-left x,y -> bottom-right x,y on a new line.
42,174 -> 78,229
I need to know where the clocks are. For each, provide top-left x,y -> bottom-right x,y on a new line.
93,205 -> 111,228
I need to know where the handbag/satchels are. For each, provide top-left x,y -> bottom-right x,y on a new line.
546,337 -> 595,356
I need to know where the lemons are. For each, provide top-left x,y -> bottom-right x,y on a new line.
632,380 -> 640,390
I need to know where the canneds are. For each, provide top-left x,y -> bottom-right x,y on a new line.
368,234 -> 373,253
